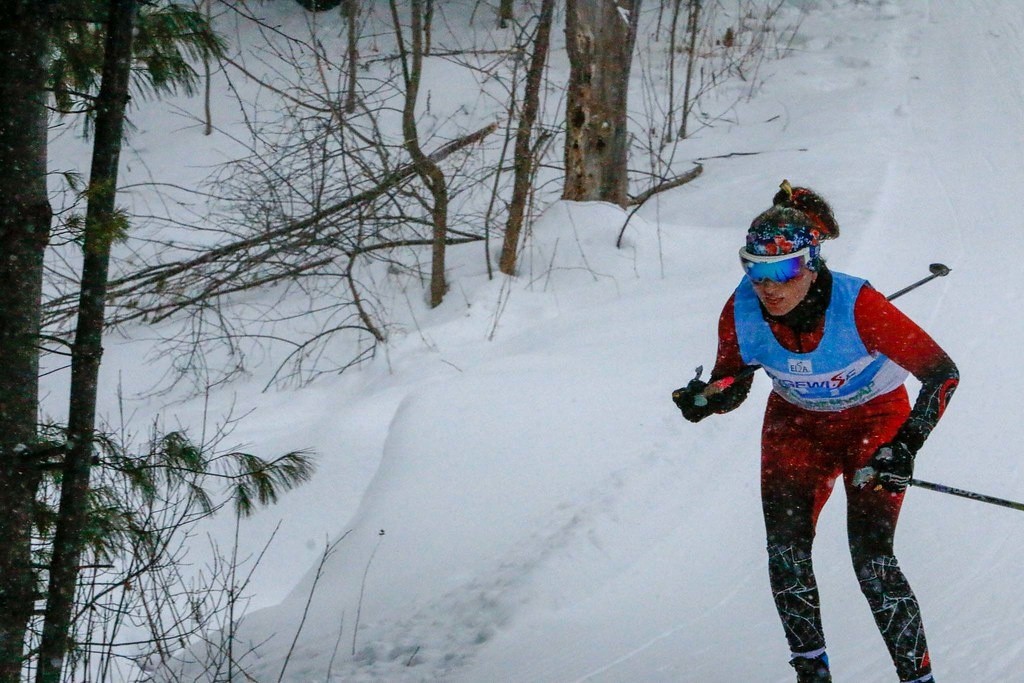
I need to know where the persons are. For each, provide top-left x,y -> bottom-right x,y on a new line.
672,180 -> 960,683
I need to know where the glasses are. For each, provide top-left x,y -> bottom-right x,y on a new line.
739,244 -> 820,284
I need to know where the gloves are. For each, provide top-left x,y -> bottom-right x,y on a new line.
672,379 -> 726,423
859,423 -> 924,493
